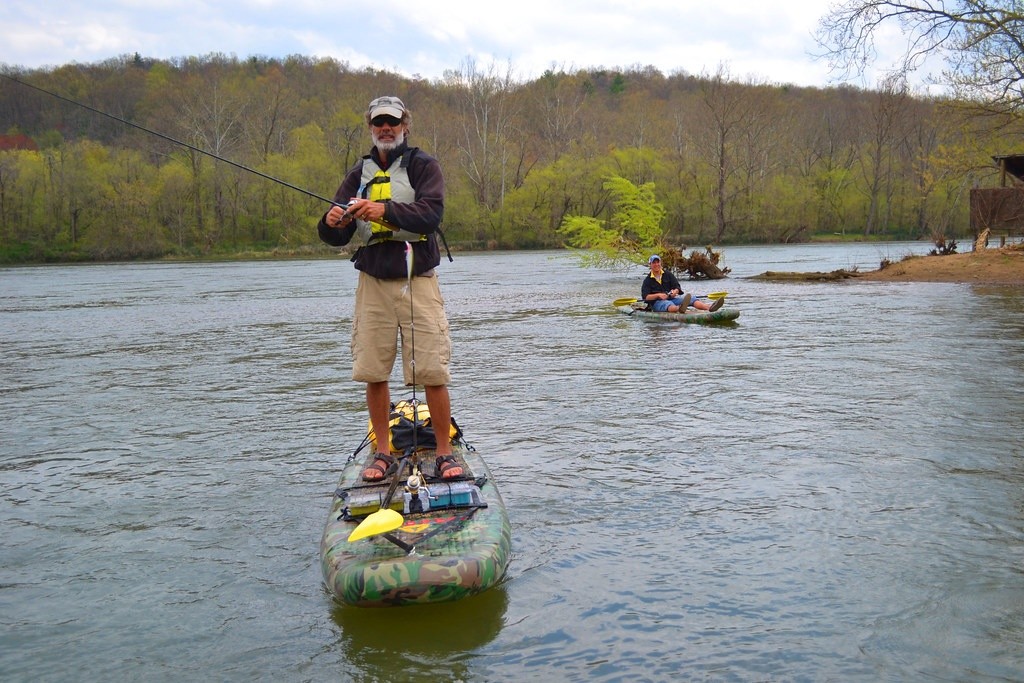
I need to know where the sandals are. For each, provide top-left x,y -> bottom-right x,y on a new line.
362,453 -> 398,482
434,454 -> 466,481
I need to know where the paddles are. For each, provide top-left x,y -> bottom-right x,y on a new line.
346,446 -> 416,542
613,291 -> 727,308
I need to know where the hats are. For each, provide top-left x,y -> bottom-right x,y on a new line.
649,255 -> 661,263
367,96 -> 406,120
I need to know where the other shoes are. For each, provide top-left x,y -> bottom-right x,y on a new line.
709,296 -> 724,312
679,293 -> 691,313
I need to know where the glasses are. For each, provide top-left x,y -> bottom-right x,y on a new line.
373,116 -> 402,127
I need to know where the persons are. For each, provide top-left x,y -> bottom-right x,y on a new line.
317,96 -> 463,480
641,255 -> 724,314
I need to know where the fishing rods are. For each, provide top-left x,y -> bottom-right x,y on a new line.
0,75 -> 401,232
